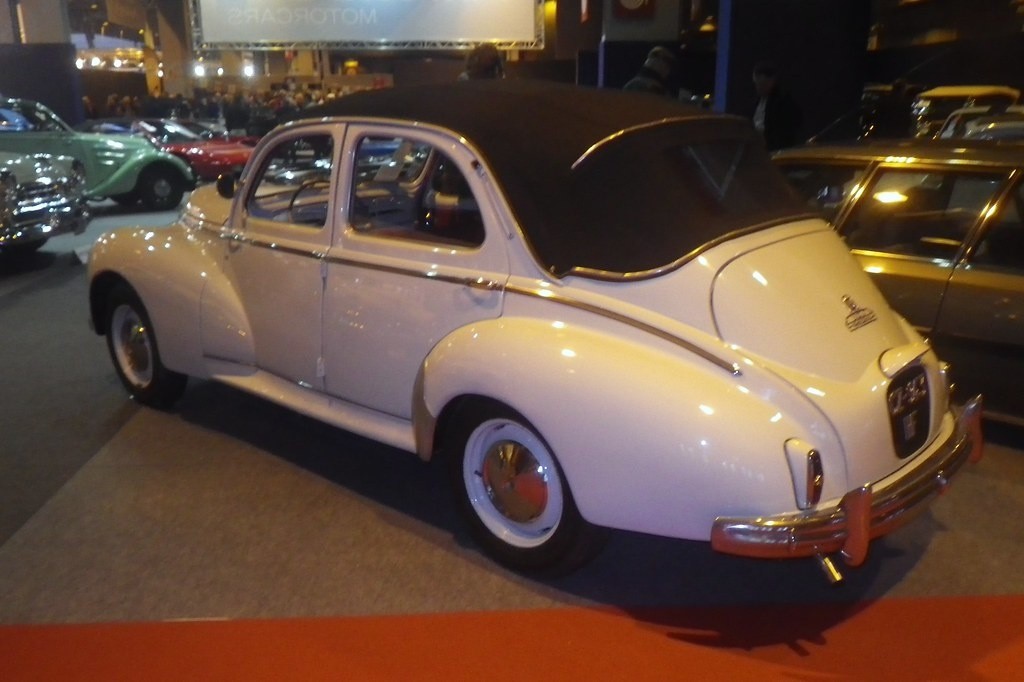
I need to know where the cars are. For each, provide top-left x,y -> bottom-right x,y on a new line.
762,42 -> 1024,434
0,96 -> 265,257
86,73 -> 985,585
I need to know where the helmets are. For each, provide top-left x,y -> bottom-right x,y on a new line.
648,46 -> 673,60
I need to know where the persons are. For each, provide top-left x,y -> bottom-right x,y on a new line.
621,44 -> 678,96
749,61 -> 803,155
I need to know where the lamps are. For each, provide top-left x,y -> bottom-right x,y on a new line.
75,22 -> 165,78
